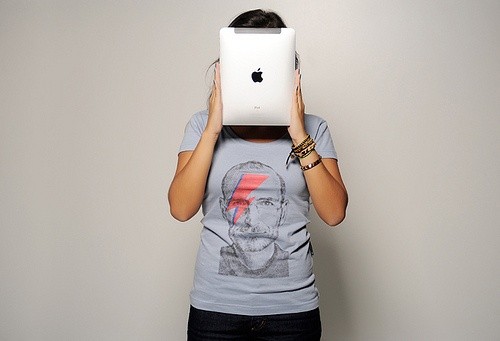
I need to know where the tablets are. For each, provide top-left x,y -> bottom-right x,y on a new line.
220,27 -> 295,126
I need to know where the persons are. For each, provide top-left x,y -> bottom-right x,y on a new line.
165,8 -> 352,341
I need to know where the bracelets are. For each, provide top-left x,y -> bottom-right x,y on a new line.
285,134 -> 317,166
300,154 -> 323,172
